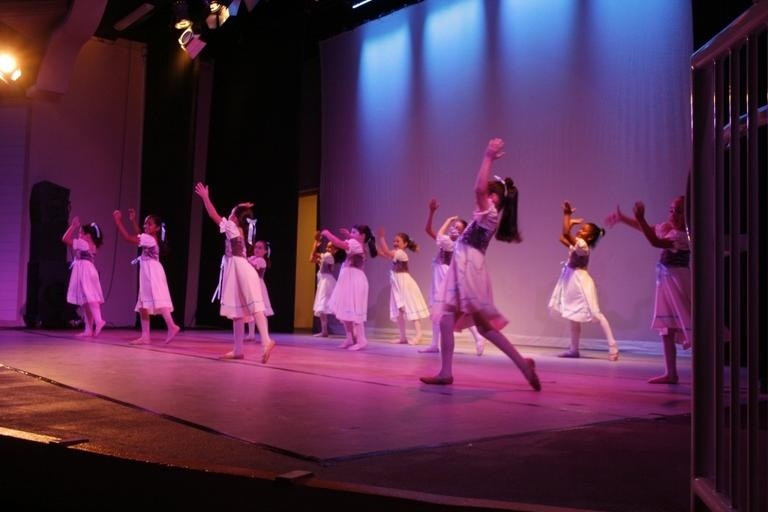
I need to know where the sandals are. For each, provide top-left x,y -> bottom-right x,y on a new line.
348,341 -> 368,350
218,352 -> 244,359
130,338 -> 151,345
648,374 -> 679,384
337,339 -> 354,348
313,332 -> 328,337
608,344 -> 619,361
525,358 -> 542,391
166,326 -> 181,344
262,340 -> 276,363
94,320 -> 106,335
418,345 -> 440,353
558,350 -> 579,357
475,336 -> 484,356
420,375 -> 453,384
390,338 -> 408,344
243,337 -> 256,344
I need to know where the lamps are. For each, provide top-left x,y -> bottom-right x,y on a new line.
0,51 -> 24,85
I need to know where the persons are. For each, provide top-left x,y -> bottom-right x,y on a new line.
113,208 -> 180,344
604,196 -> 691,385
195,182 -> 275,364
321,222 -> 377,352
374,224 -> 430,344
308,231 -> 346,336
547,200 -> 618,362
418,199 -> 484,356
242,240 -> 271,342
420,137 -> 541,392
63,215 -> 106,338
